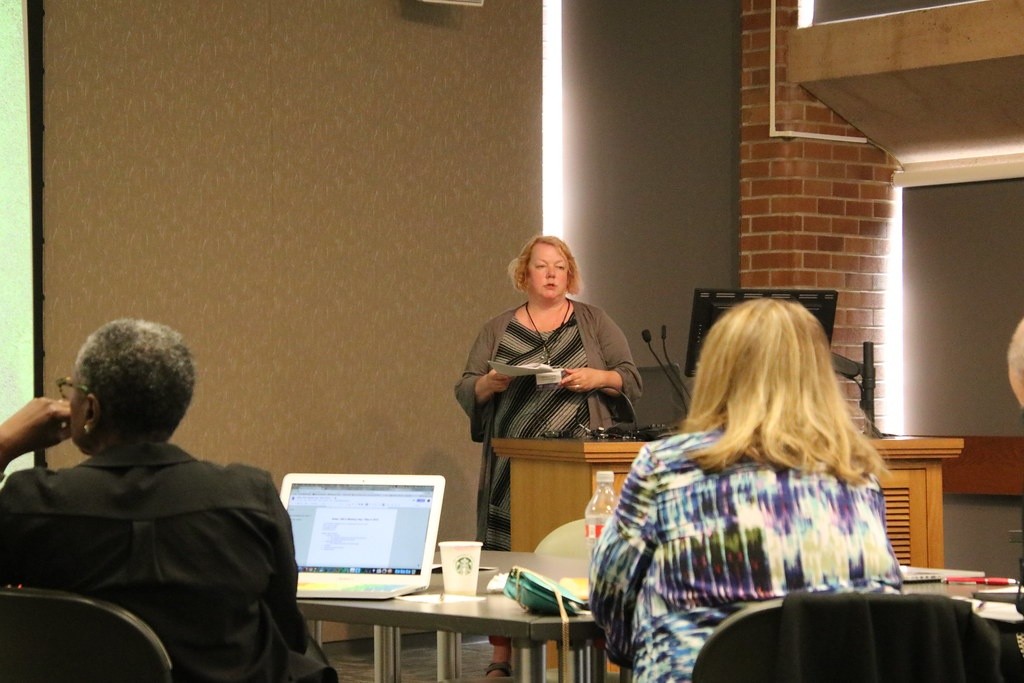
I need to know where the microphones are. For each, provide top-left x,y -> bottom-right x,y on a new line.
642,326 -> 691,415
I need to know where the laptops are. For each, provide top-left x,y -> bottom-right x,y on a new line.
281,472 -> 447,600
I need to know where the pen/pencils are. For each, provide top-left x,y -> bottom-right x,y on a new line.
941,576 -> 1020,585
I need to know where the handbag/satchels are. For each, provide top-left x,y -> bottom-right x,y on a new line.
503,566 -> 586,617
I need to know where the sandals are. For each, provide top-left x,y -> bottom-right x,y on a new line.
485,662 -> 512,678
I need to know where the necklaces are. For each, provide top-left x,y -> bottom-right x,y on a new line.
541,335 -> 549,359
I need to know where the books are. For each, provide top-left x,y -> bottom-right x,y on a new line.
900,566 -> 984,581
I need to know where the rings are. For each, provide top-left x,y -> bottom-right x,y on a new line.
577,385 -> 579,389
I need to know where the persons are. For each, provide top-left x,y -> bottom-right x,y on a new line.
589,299 -> 903,683
1007,319 -> 1024,406
0,318 -> 338,683
455,237 -> 644,676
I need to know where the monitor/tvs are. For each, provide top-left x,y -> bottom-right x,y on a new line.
684,288 -> 839,378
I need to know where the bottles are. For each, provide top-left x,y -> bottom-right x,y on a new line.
584,469 -> 619,562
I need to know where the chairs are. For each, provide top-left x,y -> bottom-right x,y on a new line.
690,590 -> 1005,683
0,587 -> 173,683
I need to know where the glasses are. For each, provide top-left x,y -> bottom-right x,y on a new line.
55,376 -> 88,399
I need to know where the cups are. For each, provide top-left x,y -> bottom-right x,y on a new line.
437,540 -> 483,597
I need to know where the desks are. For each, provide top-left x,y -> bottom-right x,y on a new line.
296,548 -> 1024,683
